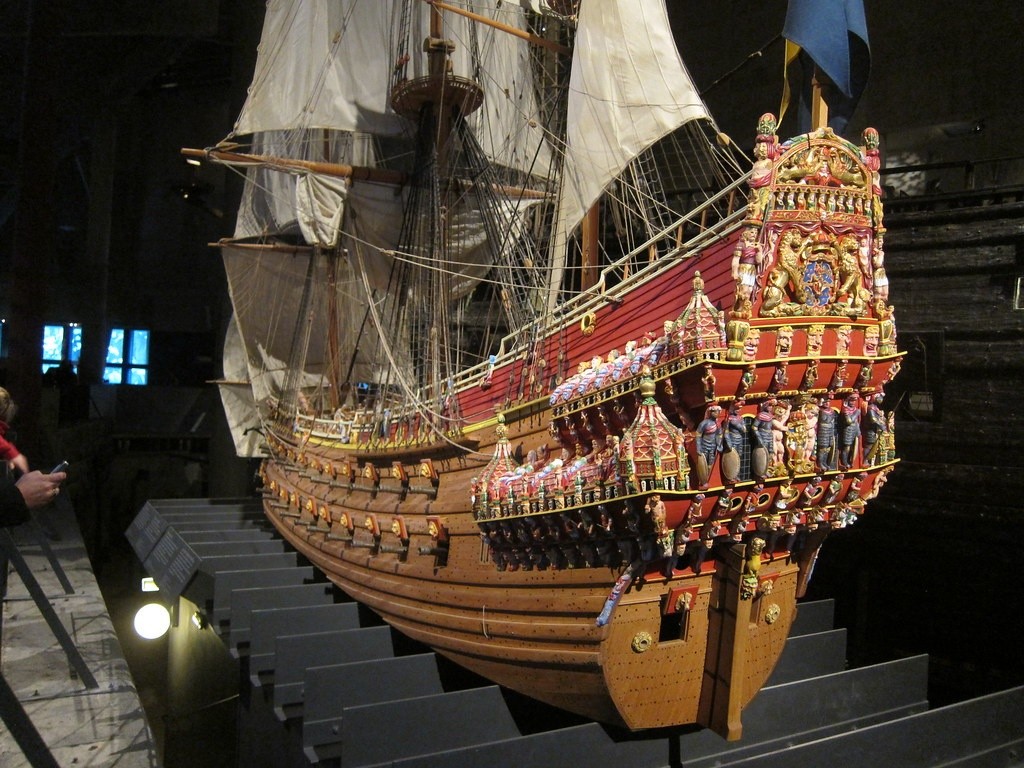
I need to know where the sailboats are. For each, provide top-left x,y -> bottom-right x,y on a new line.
183,0 -> 910,742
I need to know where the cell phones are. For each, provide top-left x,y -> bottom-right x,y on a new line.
51,461 -> 68,475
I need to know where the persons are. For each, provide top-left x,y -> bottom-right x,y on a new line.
468,320 -> 897,578
731,227 -> 762,309
0,386 -> 31,471
0,458 -> 71,526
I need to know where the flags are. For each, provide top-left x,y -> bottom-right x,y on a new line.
774,0 -> 871,141
